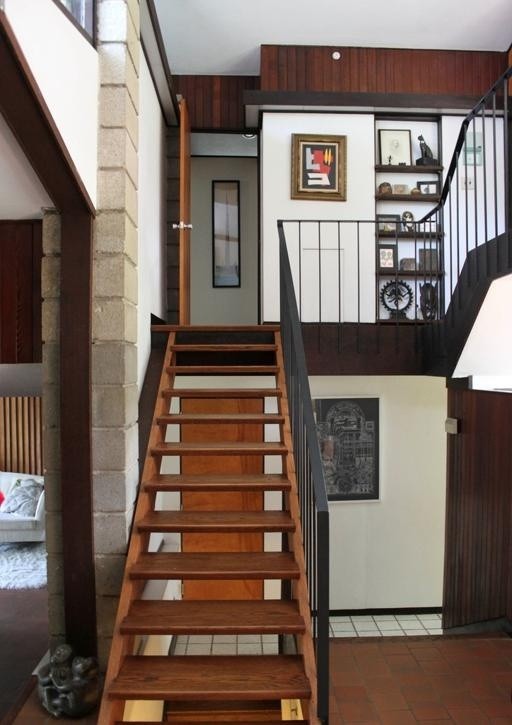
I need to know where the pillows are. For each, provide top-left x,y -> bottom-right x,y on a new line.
1,479 -> 44,517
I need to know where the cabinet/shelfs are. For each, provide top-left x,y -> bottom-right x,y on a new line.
375,116 -> 444,324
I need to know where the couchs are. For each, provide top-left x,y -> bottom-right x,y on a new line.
0,469 -> 47,545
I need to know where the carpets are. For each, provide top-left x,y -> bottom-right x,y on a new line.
0,544 -> 48,589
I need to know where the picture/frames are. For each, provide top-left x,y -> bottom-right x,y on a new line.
289,132 -> 350,204
211,179 -> 242,289
379,244 -> 398,270
377,214 -> 401,232
310,394 -> 384,505
418,181 -> 440,196
